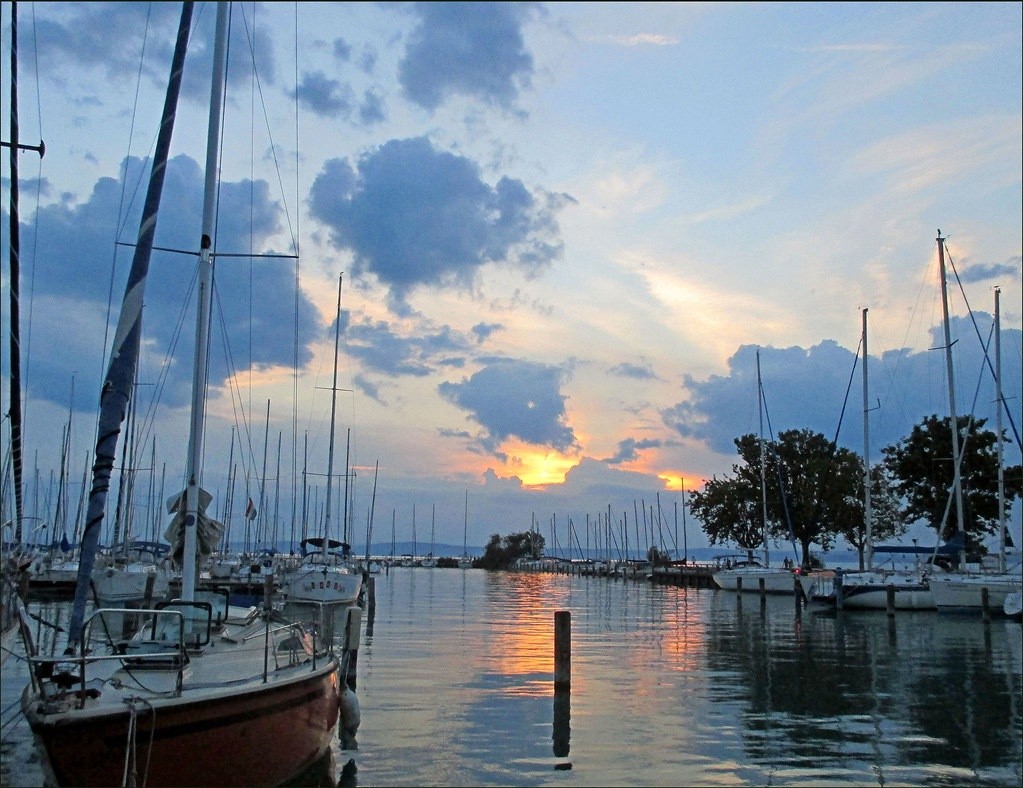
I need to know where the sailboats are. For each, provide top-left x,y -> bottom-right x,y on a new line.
508,226 -> 1021,618
2,2 -> 472,788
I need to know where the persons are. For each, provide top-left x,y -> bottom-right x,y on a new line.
692,556 -> 696,567
730,555 -> 737,568
789,559 -> 794,569
727,558 -> 732,571
716,557 -> 721,572
782,556 -> 789,570
722,558 -> 727,572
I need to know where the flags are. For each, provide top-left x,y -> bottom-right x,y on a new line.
244,498 -> 257,522
